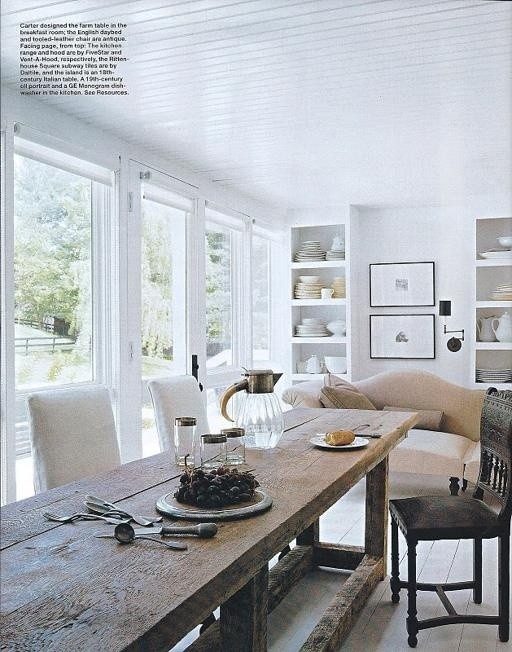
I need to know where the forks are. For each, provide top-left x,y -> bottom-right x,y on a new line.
44,495 -> 163,527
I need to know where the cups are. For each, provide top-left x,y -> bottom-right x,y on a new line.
220,426 -> 246,466
320,288 -> 335,299
173,417 -> 197,466
199,434 -> 229,470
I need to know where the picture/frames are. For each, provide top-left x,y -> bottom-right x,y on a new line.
370,314 -> 435,359
370,261 -> 435,307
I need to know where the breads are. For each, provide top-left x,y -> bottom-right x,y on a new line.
324,430 -> 356,446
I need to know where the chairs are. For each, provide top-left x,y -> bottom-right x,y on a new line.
26,380 -> 216,634
148,374 -> 291,561
389,387 -> 512,648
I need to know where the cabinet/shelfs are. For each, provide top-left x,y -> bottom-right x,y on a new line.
290,222 -> 349,384
475,216 -> 512,390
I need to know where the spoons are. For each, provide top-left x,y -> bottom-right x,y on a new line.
344,424 -> 369,433
114,523 -> 188,552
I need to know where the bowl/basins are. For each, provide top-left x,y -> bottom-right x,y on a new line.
496,235 -> 512,250
296,275 -> 322,283
324,355 -> 348,374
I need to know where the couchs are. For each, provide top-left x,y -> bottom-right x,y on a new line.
281,368 -> 487,496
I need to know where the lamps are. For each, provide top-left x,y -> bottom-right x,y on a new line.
439,301 -> 465,353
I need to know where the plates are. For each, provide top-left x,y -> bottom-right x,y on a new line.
487,282 -> 511,301
330,276 -> 345,298
478,251 -> 512,258
294,316 -> 328,338
310,436 -> 369,450
475,368 -> 512,382
294,239 -> 326,263
156,488 -> 273,522
294,283 -> 324,300
326,249 -> 345,261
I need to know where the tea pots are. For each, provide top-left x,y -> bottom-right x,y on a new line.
327,315 -> 346,337
476,314 -> 496,342
221,366 -> 287,451
305,354 -> 323,374
332,233 -> 345,252
491,311 -> 512,342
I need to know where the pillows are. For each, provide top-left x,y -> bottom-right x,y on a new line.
319,373 -> 376,410
383,404 -> 446,433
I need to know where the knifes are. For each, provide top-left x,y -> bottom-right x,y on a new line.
94,520 -> 218,539
354,433 -> 382,437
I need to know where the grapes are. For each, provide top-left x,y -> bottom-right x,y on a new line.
175,466 -> 257,507
